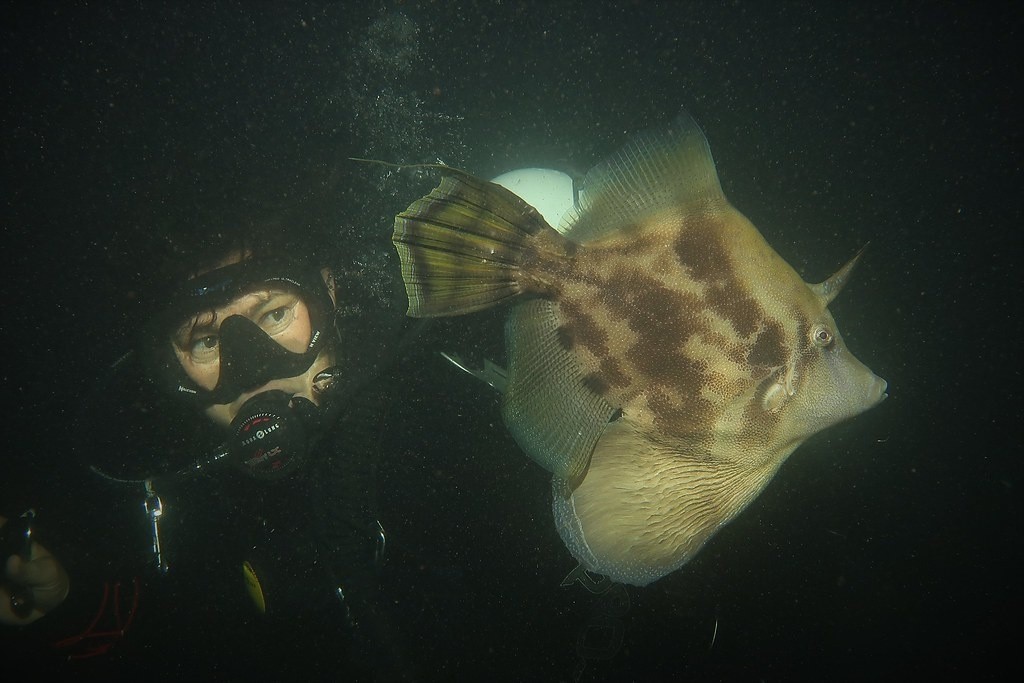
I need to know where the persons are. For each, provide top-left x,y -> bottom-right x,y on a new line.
0,176 -> 405,625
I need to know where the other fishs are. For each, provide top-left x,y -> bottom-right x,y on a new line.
392,111 -> 888,588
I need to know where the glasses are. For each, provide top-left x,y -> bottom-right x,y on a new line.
138,254 -> 336,405
